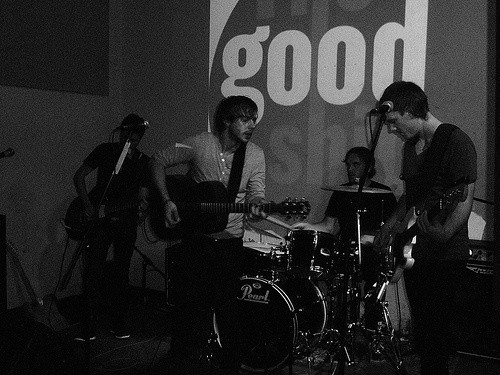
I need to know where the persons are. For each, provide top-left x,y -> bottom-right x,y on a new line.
372,79 -> 478,354
287,146 -> 398,330
72,114 -> 155,342
150,95 -> 272,346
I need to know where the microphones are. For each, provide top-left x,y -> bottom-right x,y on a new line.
370,100 -> 394,117
0,147 -> 15,159
117,121 -> 149,131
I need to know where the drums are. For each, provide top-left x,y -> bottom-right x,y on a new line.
243,242 -> 284,272
214,272 -> 326,366
288,230 -> 337,272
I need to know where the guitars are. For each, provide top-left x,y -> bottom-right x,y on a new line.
385,182 -> 465,284
64,197 -> 140,240
149,175 -> 311,241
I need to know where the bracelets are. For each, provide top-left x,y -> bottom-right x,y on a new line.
163,199 -> 172,206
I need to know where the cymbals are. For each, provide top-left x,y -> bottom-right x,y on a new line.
245,223 -> 281,240
322,185 -> 392,193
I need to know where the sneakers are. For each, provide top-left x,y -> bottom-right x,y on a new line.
72,327 -> 97,342
110,323 -> 145,339
